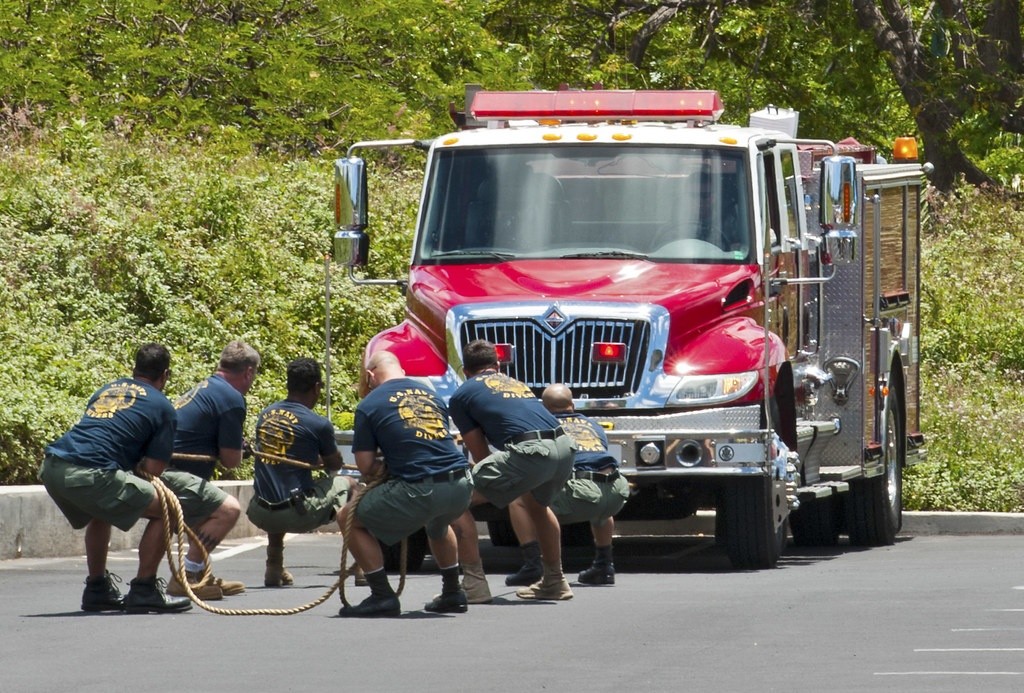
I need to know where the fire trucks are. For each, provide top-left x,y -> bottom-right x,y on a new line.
330,83 -> 925,570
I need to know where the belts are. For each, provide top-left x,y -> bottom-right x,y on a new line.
405,467 -> 469,484
568,469 -> 620,483
509,427 -> 565,445
255,492 -> 315,511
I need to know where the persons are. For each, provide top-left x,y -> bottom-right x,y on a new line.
339,350 -> 474,617
505,383 -> 629,587
39,342 -> 193,615
247,358 -> 371,588
663,171 -> 741,252
432,339 -> 574,604
159,339 -> 260,600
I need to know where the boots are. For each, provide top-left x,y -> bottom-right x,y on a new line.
125,574 -> 193,613
81,570 -> 126,613
578,543 -> 615,585
264,546 -> 293,588
460,563 -> 492,605
339,569 -> 401,618
166,569 -> 246,601
516,559 -> 573,601
354,565 -> 370,586
506,544 -> 544,587
425,563 -> 468,614
